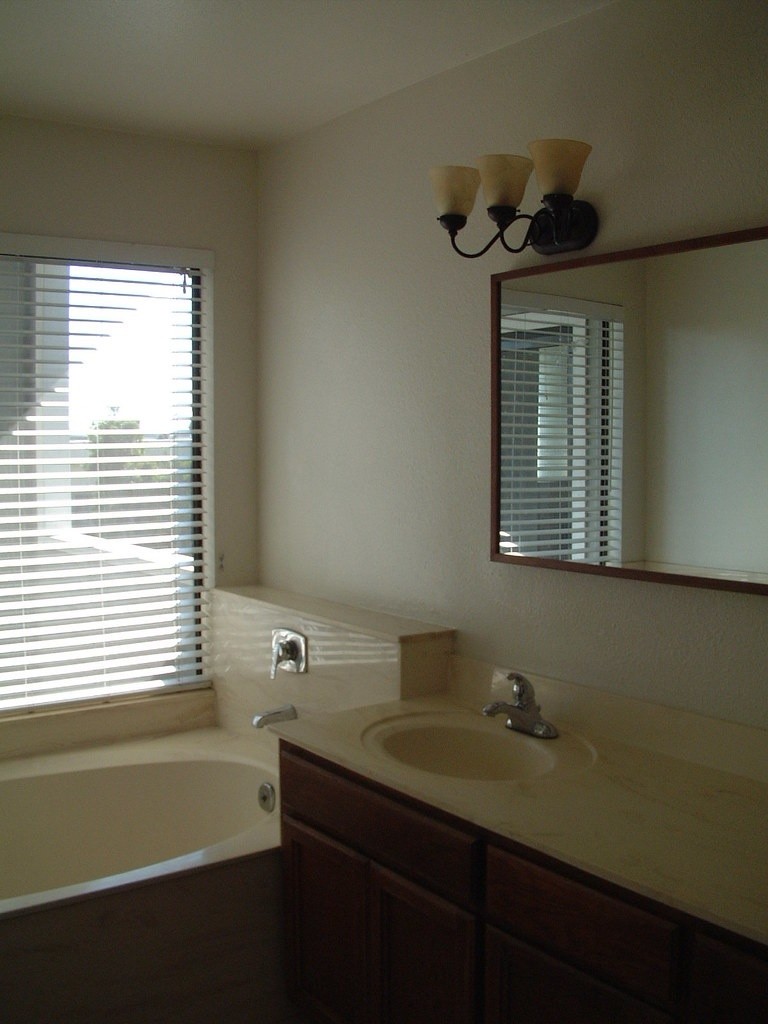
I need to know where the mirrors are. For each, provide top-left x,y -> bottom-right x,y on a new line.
490,226 -> 768,595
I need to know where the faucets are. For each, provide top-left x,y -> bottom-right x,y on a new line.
250,703 -> 297,728
479,671 -> 559,740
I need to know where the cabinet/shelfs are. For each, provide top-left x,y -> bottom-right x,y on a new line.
279,739 -> 768,1024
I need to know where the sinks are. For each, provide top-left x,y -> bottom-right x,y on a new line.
360,710 -> 597,792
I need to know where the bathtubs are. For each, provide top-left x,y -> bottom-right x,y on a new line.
0,721 -> 280,1024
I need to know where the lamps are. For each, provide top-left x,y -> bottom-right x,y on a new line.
430,139 -> 599,258
